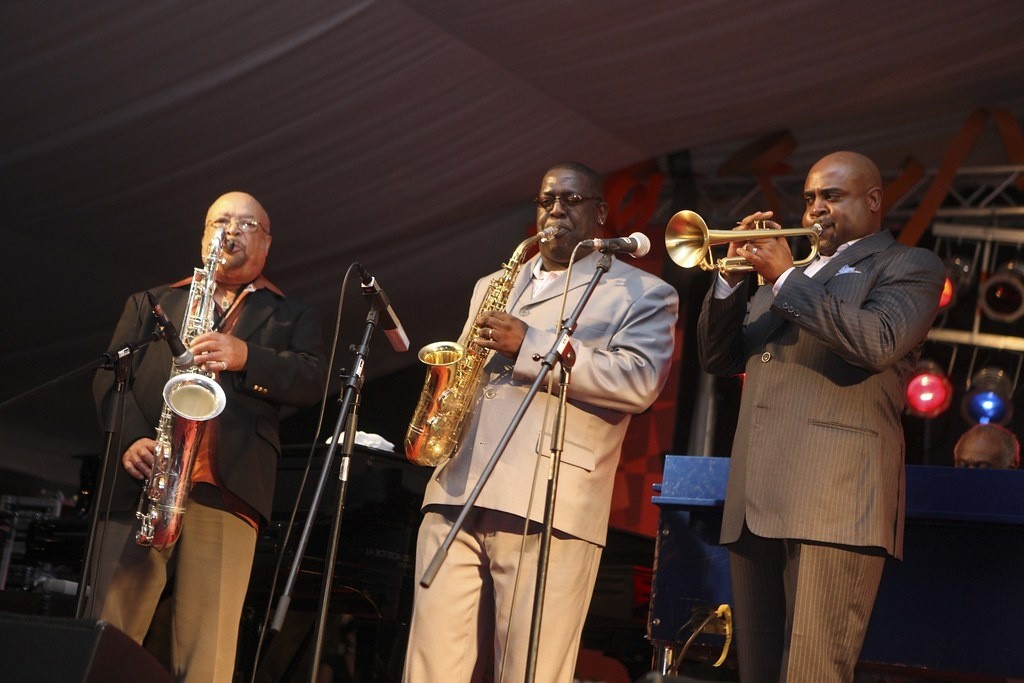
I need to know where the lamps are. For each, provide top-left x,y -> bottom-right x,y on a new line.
907,222 -> 1024,467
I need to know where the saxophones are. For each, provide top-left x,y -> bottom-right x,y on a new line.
135,224 -> 227,551
405,226 -> 551,468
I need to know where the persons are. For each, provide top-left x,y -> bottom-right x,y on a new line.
952,422 -> 1021,469
402,161 -> 680,683
83,192 -> 325,683
698,150 -> 947,683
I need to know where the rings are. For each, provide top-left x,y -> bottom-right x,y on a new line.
223,362 -> 227,370
752,248 -> 758,254
489,329 -> 493,339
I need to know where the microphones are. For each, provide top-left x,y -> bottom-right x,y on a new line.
578,232 -> 650,259
355,263 -> 410,352
147,293 -> 194,370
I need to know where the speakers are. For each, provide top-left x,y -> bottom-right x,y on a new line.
0,612 -> 178,683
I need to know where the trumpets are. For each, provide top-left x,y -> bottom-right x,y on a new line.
664,208 -> 822,272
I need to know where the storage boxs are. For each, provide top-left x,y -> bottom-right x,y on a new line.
274,443 -> 437,526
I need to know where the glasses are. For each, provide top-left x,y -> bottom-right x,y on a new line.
207,217 -> 270,234
531,194 -> 602,206
